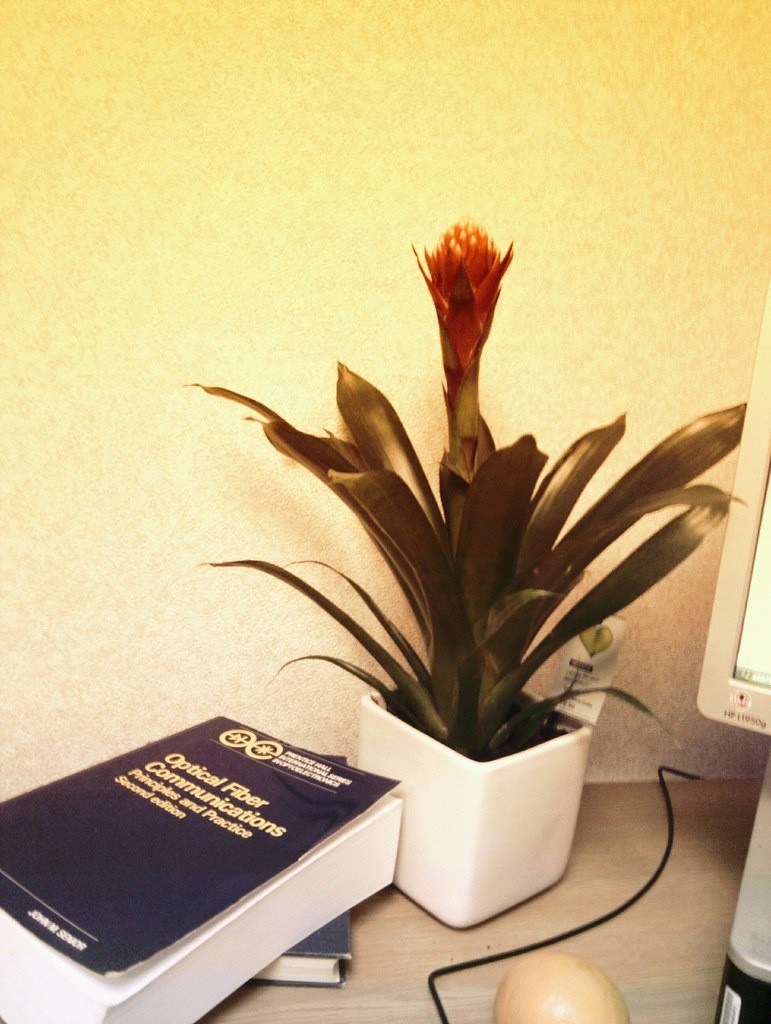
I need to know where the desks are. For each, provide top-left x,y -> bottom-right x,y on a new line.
200,781 -> 761,1024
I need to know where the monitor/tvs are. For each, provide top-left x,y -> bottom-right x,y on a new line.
696,272 -> 771,983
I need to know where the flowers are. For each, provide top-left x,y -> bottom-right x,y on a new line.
178,218 -> 746,758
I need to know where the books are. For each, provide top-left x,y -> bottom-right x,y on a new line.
0,716 -> 404,1024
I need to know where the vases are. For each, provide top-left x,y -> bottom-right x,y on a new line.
358,674 -> 592,929
